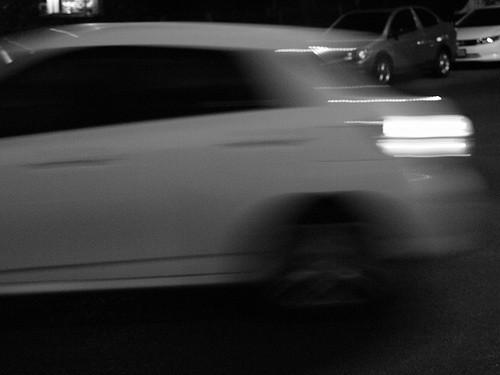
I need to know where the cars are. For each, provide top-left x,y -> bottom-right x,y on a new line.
307,7 -> 460,83
0,20 -> 482,322
453,6 -> 500,65
